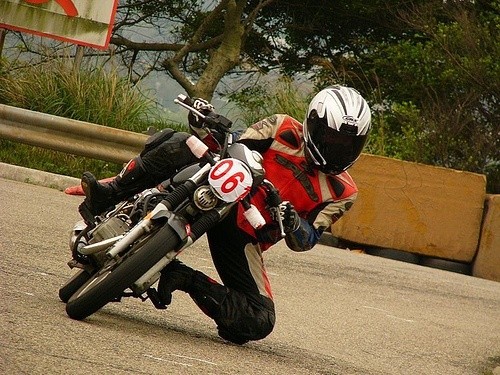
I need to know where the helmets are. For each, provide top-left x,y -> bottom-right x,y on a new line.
303,84 -> 372,176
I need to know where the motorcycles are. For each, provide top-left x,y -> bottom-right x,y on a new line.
58,93 -> 287,321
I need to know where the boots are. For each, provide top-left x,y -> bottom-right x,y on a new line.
81,154 -> 155,215
157,258 -> 228,319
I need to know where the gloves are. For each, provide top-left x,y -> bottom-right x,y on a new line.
266,200 -> 299,235
187,98 -> 214,127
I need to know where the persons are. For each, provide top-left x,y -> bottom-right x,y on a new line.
81,83 -> 372,346
